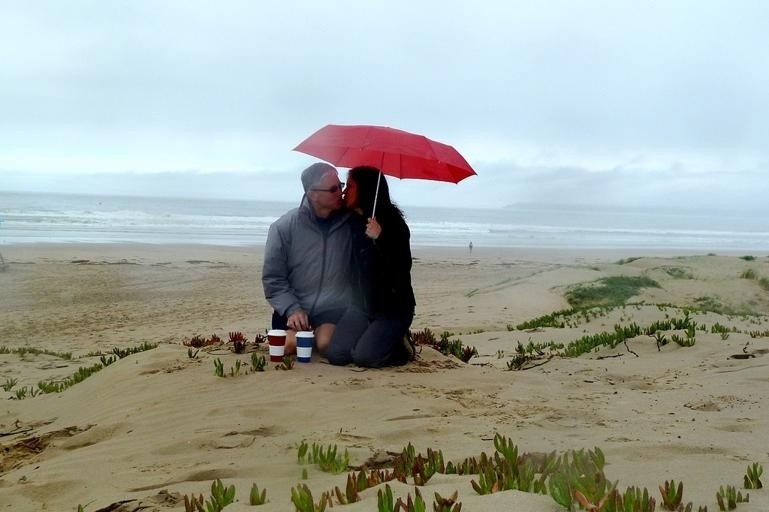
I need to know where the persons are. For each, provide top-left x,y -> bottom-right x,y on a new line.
261,163 -> 357,355
327,165 -> 416,368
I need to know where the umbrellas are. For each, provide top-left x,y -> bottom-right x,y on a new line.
290,124 -> 478,219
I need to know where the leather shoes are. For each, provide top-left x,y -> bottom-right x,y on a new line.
403,333 -> 415,363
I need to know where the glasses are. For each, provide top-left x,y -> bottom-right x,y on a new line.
310,183 -> 345,193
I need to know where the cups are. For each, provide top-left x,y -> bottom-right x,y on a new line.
268,329 -> 287,363
295,329 -> 316,362
